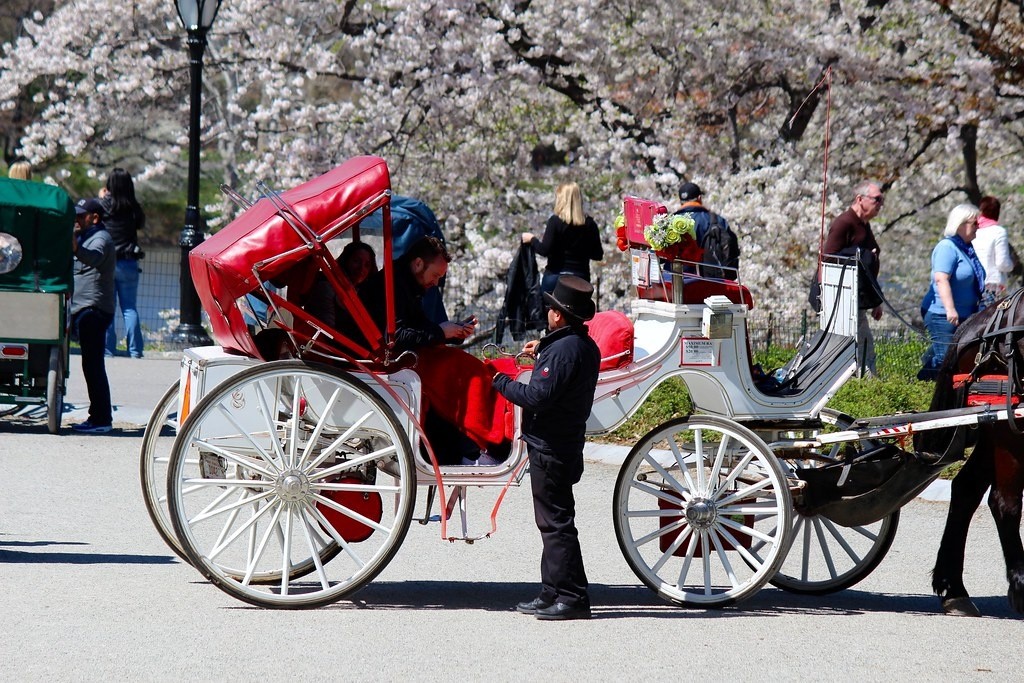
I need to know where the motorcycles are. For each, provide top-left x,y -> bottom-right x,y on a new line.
0,172 -> 78,432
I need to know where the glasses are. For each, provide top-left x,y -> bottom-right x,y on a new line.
964,221 -> 980,226
861,194 -> 885,203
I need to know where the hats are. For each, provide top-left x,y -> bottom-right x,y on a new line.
74,198 -> 105,218
678,182 -> 705,204
542,274 -> 596,321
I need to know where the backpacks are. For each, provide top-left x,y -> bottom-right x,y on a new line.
699,212 -> 740,281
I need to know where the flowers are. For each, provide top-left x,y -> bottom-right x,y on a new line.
613,205 -> 705,266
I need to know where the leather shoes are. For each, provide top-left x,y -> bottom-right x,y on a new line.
534,602 -> 591,619
517,597 -> 553,613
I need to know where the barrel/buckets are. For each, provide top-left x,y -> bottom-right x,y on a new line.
657,453 -> 756,557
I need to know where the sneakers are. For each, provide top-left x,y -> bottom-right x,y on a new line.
72,420 -> 113,432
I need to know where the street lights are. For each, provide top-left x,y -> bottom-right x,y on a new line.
170,0 -> 225,354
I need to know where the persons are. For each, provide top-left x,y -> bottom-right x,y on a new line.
7,160 -> 32,180
521,181 -> 603,304
650,182 -> 740,281
490,276 -> 601,620
94,169 -> 146,359
69,198 -> 116,434
305,234 -> 474,370
817,182 -> 882,381
922,197 -> 1016,365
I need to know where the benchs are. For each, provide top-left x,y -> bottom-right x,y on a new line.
266,299 -> 430,459
623,195 -> 754,312
0,284 -> 70,346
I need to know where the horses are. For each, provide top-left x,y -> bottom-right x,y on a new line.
912,288 -> 1024,618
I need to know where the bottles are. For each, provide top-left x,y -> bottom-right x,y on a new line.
775,368 -> 787,382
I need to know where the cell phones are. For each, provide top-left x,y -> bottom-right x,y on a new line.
461,319 -> 474,327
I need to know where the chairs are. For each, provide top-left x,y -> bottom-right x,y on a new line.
481,310 -> 636,429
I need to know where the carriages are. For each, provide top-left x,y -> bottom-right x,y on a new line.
135,150 -> 1024,626
